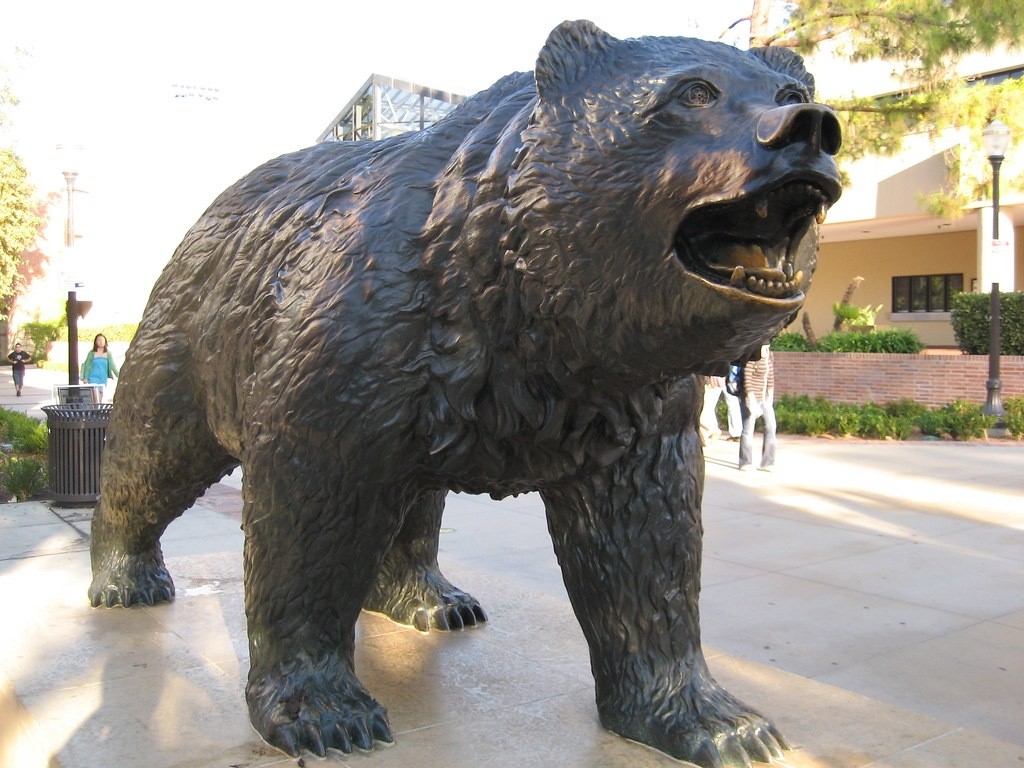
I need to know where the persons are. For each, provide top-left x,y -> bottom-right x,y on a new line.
7,343 -> 31,397
84,333 -> 119,404
701,338 -> 781,474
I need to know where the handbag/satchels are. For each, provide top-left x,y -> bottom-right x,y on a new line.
726,365 -> 743,396
79,363 -> 85,381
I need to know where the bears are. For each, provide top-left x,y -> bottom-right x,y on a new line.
90,18 -> 846,766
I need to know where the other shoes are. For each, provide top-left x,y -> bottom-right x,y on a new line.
759,465 -> 775,472
733,437 -> 741,442
17,391 -> 21,396
740,464 -> 753,471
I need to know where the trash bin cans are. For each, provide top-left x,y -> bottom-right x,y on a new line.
41,402 -> 114,508
